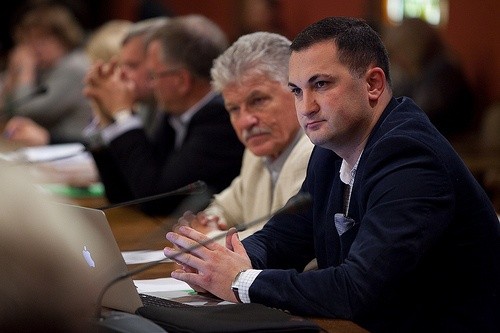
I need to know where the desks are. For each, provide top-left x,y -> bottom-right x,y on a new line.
87,205 -> 367,333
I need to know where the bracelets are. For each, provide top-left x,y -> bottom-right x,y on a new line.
109,110 -> 138,123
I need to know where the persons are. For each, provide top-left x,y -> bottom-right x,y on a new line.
0,3 -> 171,188
163,17 -> 500,333
172,31 -> 318,272
79,13 -> 246,219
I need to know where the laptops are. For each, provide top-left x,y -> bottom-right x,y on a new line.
43,200 -> 221,316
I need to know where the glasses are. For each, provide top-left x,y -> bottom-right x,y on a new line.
148,70 -> 180,80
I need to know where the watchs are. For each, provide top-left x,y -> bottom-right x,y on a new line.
231,269 -> 246,304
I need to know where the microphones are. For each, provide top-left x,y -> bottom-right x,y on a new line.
87,191 -> 311,332
93,179 -> 208,211
0,84 -> 48,121
